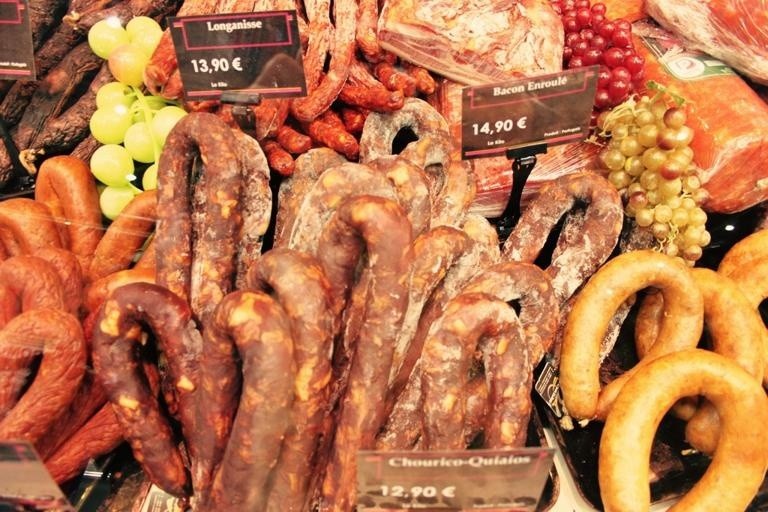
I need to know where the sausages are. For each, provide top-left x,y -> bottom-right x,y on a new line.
0,0 -> 768,512
